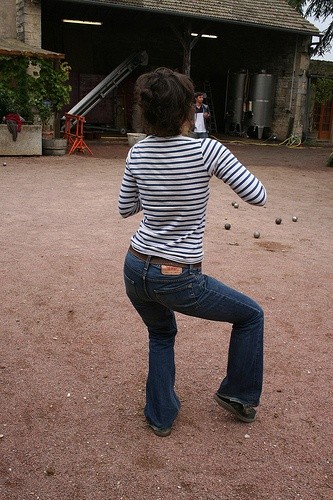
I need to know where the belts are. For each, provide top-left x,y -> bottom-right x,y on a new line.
129,244 -> 202,269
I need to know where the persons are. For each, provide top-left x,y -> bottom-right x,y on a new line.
189,92 -> 210,139
118,67 -> 267,437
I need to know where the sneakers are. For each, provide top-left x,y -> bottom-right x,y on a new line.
149,422 -> 171,436
214,390 -> 256,422
